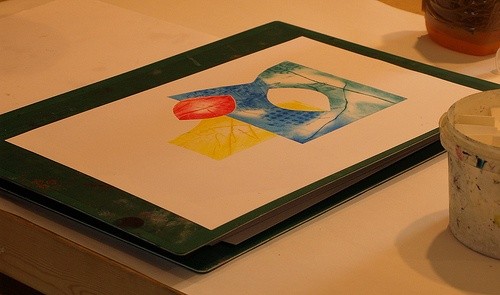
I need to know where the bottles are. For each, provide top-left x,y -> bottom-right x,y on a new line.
420,1 -> 500,57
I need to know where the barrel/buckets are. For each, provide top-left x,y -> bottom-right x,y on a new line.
438,86 -> 500,262
